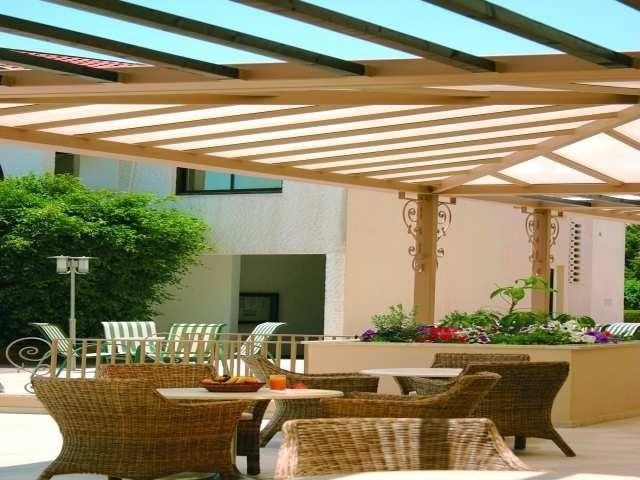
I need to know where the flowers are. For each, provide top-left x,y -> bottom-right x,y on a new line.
355,325 -> 514,344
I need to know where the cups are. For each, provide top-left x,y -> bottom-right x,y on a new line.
269,374 -> 288,391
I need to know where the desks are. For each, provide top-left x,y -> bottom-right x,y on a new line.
356,362 -> 470,386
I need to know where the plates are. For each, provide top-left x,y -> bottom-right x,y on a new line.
200,382 -> 267,392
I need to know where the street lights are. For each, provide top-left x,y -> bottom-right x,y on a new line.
46,254 -> 99,369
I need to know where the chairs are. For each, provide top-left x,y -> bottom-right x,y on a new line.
99,319 -> 162,376
463,363 -> 578,460
327,373 -> 498,426
265,413 -> 533,480
162,379 -> 341,473
224,322 -> 287,380
597,322 -> 640,337
163,321 -> 220,365
30,372 -> 242,478
30,323 -> 107,393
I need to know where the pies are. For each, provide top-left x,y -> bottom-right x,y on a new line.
201,374 -> 259,384
